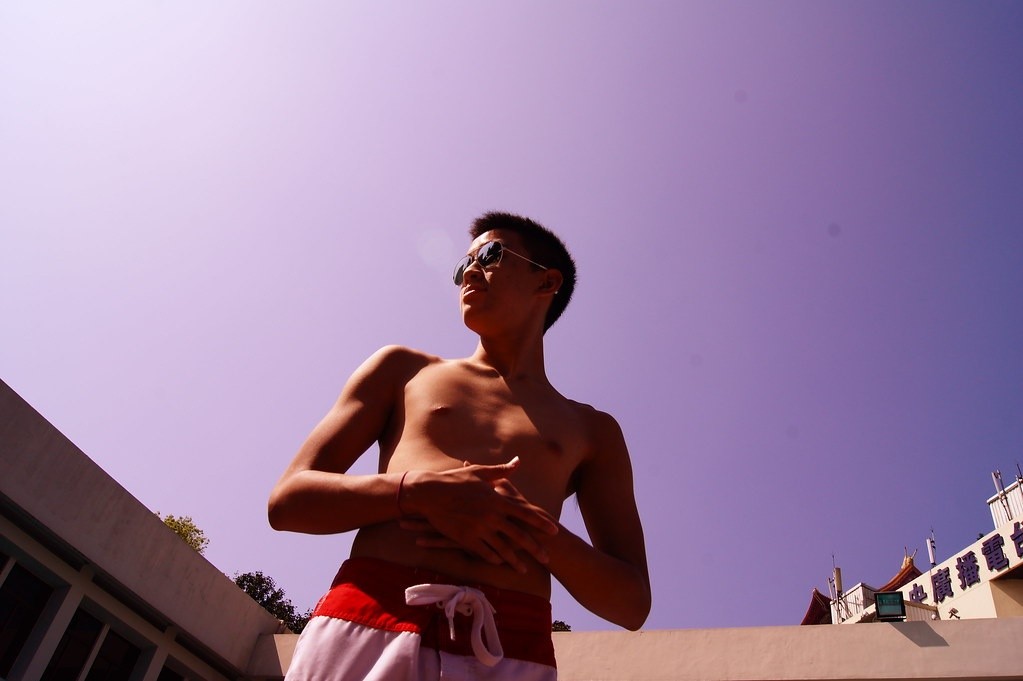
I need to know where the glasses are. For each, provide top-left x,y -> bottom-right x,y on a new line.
452,239 -> 559,295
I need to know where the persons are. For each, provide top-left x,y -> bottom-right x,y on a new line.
269,212 -> 653,681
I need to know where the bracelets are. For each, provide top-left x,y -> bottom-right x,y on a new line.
396,471 -> 409,516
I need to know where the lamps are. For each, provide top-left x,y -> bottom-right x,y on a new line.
949,608 -> 960,620
873,590 -> 908,623
